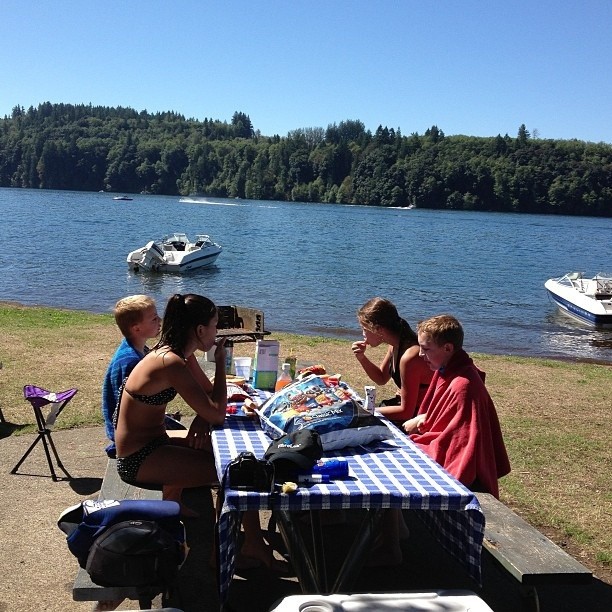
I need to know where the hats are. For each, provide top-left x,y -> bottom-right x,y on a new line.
264,429 -> 323,469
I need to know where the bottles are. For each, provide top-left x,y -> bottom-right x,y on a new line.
286,356 -> 296,379
275,362 -> 292,394
224,338 -> 234,375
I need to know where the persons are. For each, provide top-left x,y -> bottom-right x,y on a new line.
112,293 -> 289,574
351,296 -> 435,435
101,294 -> 219,561
401,313 -> 511,501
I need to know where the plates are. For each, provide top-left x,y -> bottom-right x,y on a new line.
228,402 -> 246,417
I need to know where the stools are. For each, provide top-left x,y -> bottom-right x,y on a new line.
11,383 -> 77,484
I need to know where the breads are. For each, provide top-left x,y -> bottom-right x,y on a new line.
300,364 -> 326,376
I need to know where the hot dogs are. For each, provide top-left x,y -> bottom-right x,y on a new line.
224,375 -> 245,387
240,398 -> 257,415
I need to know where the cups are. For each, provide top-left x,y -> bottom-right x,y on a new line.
234,358 -> 251,382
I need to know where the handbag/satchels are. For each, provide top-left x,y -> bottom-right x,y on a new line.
253,371 -> 395,454
86,520 -> 179,587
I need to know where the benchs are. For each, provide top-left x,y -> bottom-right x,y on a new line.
72,456 -> 163,610
470,486 -> 593,612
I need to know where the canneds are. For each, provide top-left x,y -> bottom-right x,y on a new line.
313,457 -> 348,478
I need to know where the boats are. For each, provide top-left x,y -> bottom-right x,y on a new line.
112,194 -> 133,201
127,232 -> 223,274
545,270 -> 612,330
